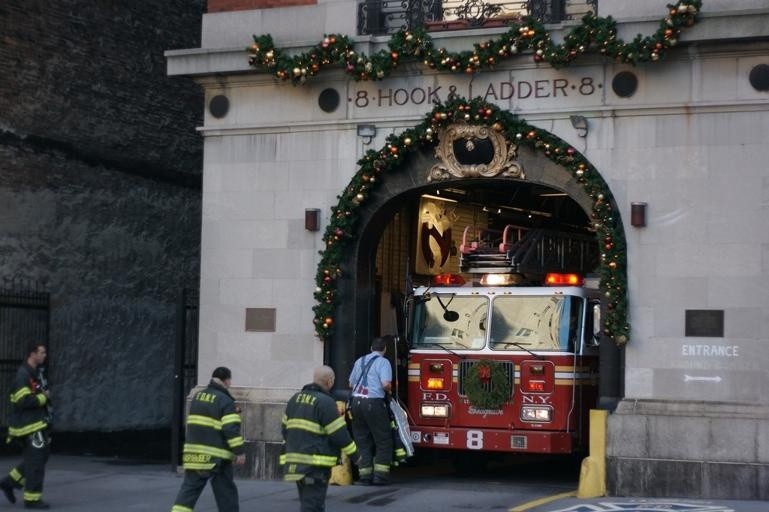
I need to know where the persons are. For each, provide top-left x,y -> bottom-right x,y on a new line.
280,364 -> 364,512
0,341 -> 53,511
348,338 -> 394,485
173,365 -> 246,511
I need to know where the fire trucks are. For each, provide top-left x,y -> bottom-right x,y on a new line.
387,224 -> 601,456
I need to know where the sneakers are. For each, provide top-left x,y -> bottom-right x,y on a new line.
362,478 -> 372,485
373,475 -> 393,485
